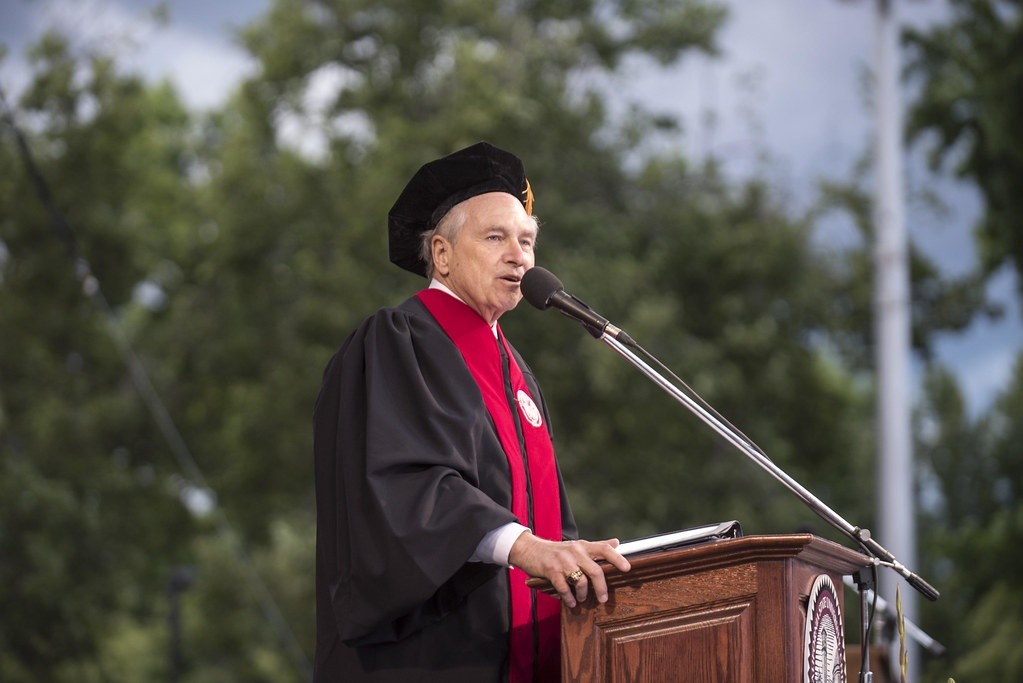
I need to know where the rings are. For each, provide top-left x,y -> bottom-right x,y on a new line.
568,571 -> 583,583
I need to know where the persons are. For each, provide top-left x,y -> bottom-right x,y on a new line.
313,142 -> 632,683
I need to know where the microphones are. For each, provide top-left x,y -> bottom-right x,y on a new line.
520,266 -> 636,347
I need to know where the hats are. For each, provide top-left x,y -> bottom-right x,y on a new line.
387,141 -> 535,280
